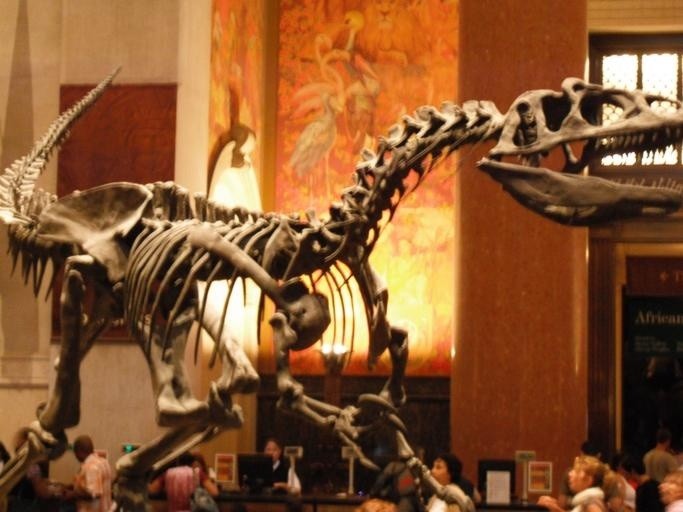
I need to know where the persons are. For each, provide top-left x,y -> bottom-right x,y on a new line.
537,434 -> 683,512
60,434 -> 113,512
262,435 -> 302,495
146,449 -> 222,512
366,446 -> 483,512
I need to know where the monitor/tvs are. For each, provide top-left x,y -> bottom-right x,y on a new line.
236,452 -> 274,494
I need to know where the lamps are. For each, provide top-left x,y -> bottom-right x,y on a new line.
220,125 -> 257,168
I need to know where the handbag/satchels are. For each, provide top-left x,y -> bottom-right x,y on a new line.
188,487 -> 219,512
